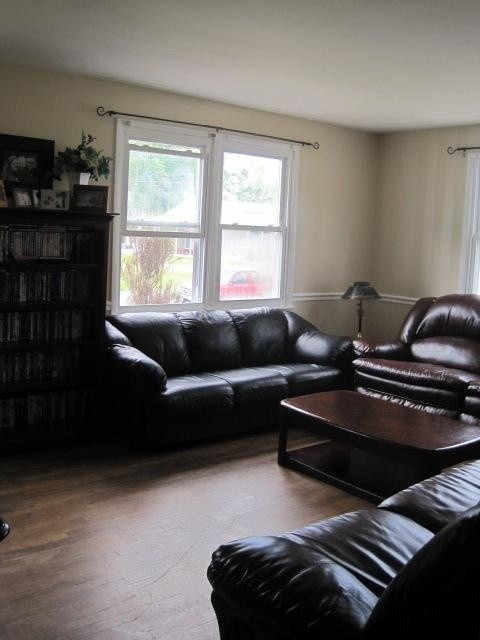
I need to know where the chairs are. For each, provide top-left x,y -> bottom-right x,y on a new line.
353,293 -> 480,430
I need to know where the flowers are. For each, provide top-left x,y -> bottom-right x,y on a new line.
50,126 -> 114,182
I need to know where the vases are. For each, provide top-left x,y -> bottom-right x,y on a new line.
68,172 -> 91,199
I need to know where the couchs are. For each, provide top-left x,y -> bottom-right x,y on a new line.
201,455 -> 480,640
105,305 -> 355,454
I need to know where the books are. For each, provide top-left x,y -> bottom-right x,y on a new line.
1,223 -> 97,429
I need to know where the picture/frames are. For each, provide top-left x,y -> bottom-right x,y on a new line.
0,133 -> 55,184
4,180 -> 109,212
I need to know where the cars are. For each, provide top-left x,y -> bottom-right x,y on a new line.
220,270 -> 272,296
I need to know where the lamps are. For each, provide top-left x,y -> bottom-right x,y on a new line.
341,282 -> 382,337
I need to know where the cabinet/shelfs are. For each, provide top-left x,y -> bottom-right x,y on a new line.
0,206 -> 116,455
